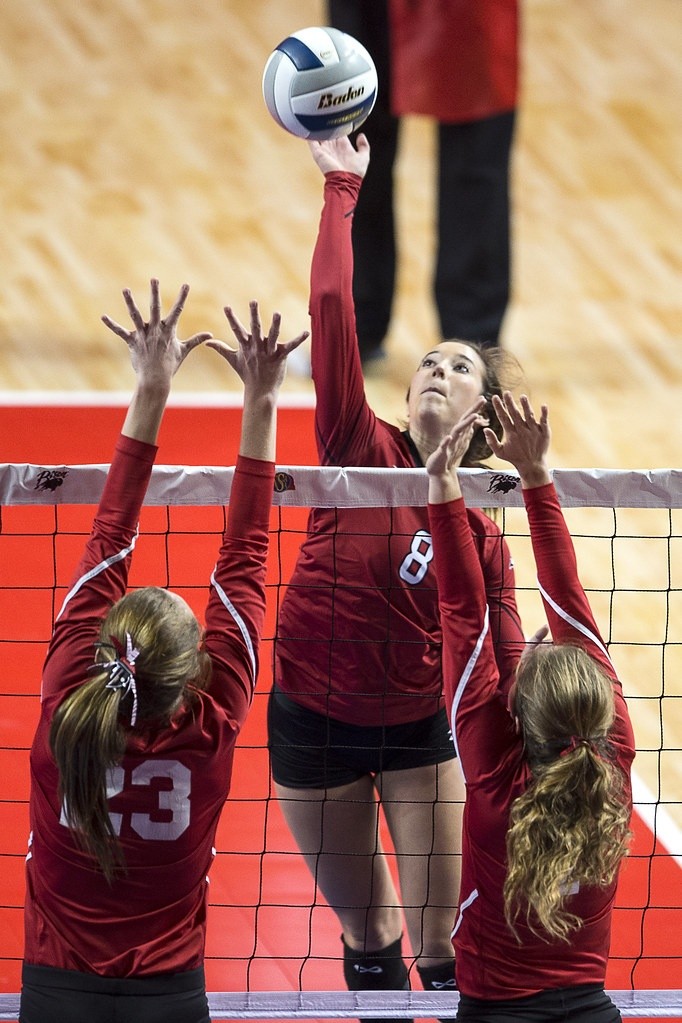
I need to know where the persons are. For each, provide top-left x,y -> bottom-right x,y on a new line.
424,389 -> 637,1023
266,135 -> 530,1023
17,278 -> 309,1023
323,0 -> 522,370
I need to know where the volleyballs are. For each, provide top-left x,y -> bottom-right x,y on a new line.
262,26 -> 378,140
